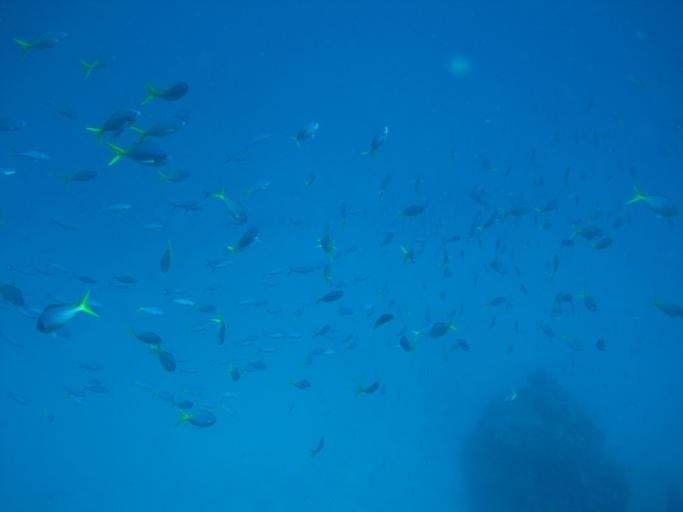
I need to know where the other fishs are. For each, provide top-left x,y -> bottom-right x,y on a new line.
0,108 -> 626,427
